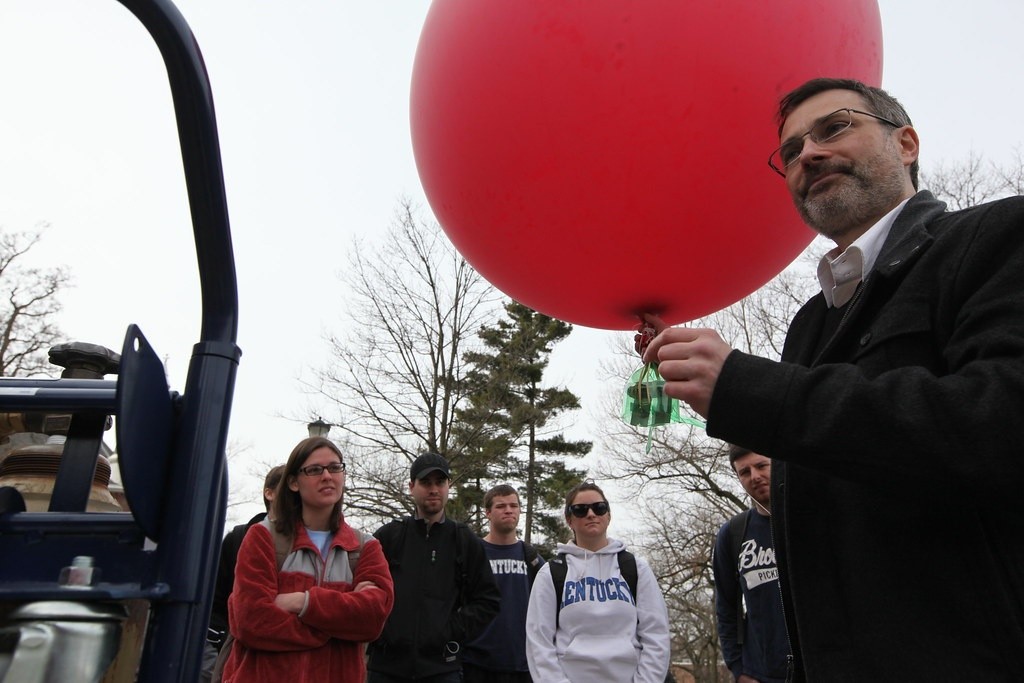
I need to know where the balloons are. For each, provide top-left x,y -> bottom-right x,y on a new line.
409,1 -> 883,360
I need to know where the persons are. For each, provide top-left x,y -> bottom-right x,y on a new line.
200,465 -> 289,683
365,453 -> 505,683
635,77 -> 1023,683
711,442 -> 799,683
525,478 -> 671,683
479,486 -> 547,683
220,436 -> 394,683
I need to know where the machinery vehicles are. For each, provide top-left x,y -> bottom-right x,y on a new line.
0,1 -> 249,683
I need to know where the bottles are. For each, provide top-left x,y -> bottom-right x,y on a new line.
57,555 -> 94,586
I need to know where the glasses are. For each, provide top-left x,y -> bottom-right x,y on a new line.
568,501 -> 609,518
300,462 -> 346,476
767,107 -> 903,180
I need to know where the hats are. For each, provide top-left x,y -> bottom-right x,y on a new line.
410,452 -> 450,481
